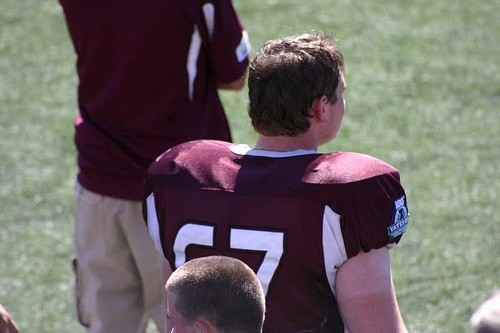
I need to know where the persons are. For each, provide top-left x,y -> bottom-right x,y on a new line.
57,0 -> 250,333
142,30 -> 412,333
164,256 -> 266,333
